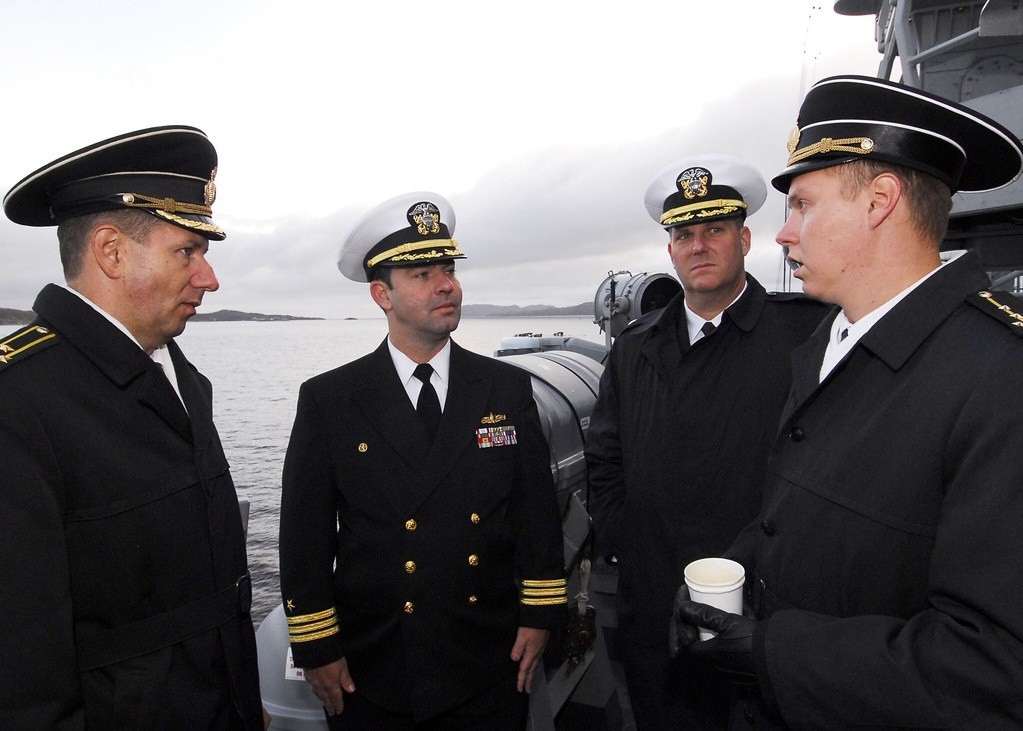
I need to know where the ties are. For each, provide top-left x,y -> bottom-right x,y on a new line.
703,321 -> 715,336
841,328 -> 850,339
412,363 -> 443,442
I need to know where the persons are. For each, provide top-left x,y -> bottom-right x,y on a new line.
584,155 -> 830,731
666,74 -> 1023,730
278,190 -> 570,731
1,125 -> 272,731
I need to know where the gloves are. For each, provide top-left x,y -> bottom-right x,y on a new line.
678,601 -> 762,697
668,579 -> 699,673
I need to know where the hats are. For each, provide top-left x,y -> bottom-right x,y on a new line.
2,124 -> 228,242
337,190 -> 469,283
643,151 -> 769,229
770,75 -> 1023,196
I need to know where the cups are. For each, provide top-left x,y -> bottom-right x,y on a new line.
684,557 -> 745,642
